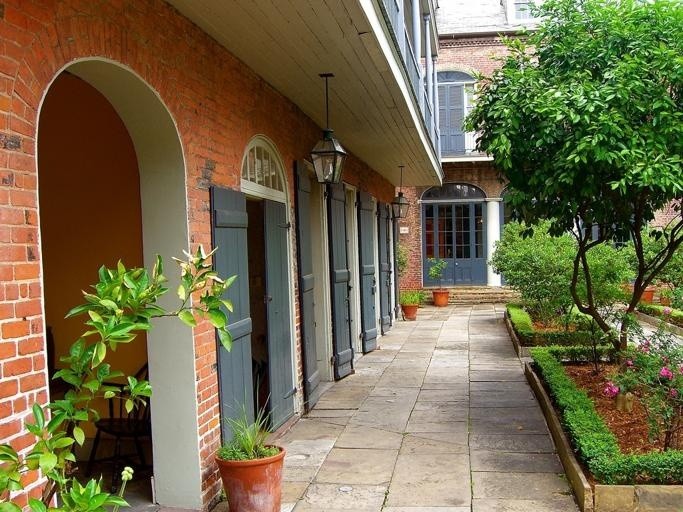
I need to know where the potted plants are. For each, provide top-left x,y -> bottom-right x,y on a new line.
398,290 -> 425,321
426,255 -> 449,307
212,374 -> 286,511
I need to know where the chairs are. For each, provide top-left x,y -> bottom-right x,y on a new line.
82,362 -> 150,497
44,326 -> 78,462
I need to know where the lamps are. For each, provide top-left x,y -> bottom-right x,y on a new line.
309,72 -> 348,184
388,166 -> 410,221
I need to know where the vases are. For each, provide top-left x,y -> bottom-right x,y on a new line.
638,284 -> 656,304
